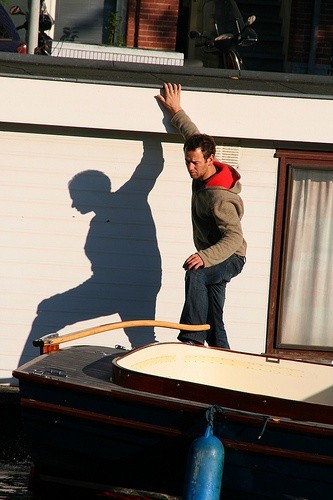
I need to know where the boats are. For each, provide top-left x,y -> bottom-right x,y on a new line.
12,330 -> 332,500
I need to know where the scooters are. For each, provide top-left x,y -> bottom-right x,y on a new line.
9,0 -> 56,55
187,14 -> 260,69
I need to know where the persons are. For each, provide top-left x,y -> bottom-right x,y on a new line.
154,82 -> 248,349
